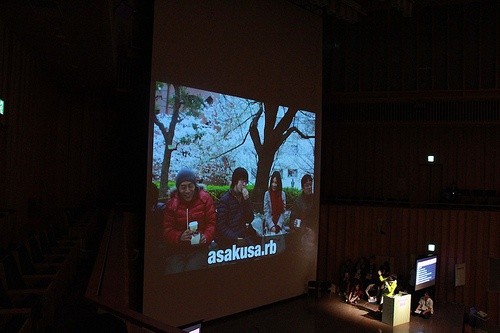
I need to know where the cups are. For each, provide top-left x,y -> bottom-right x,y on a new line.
296,220 -> 301,227
189,221 -> 198,233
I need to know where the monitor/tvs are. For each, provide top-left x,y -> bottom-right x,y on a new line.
177,318 -> 206,333
414,253 -> 438,292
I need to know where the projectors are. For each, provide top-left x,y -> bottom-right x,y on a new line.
477,310 -> 487,318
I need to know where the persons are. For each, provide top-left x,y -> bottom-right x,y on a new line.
338,255 -> 390,303
163,171 -> 218,272
263,171 -> 286,237
376,270 -> 398,313
290,175 -> 315,231
215,168 -> 261,249
414,292 -> 434,319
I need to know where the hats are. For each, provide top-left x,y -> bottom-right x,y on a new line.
175,168 -> 196,188
